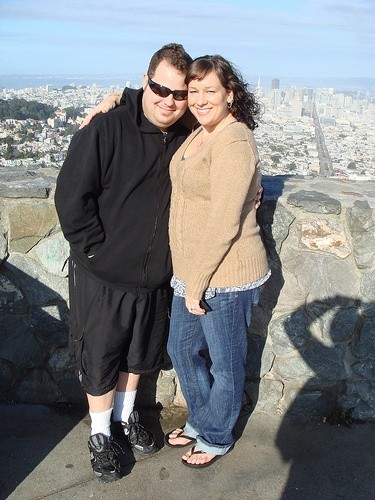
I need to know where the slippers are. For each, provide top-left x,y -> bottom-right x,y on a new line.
181,442 -> 235,468
164,424 -> 198,448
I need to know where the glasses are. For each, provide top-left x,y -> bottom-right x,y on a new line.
147,75 -> 188,101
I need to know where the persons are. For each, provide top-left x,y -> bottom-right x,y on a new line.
78,54 -> 272,468
54,43 -> 263,482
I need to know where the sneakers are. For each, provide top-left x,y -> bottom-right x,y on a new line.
112,411 -> 159,455
88,431 -> 126,482
